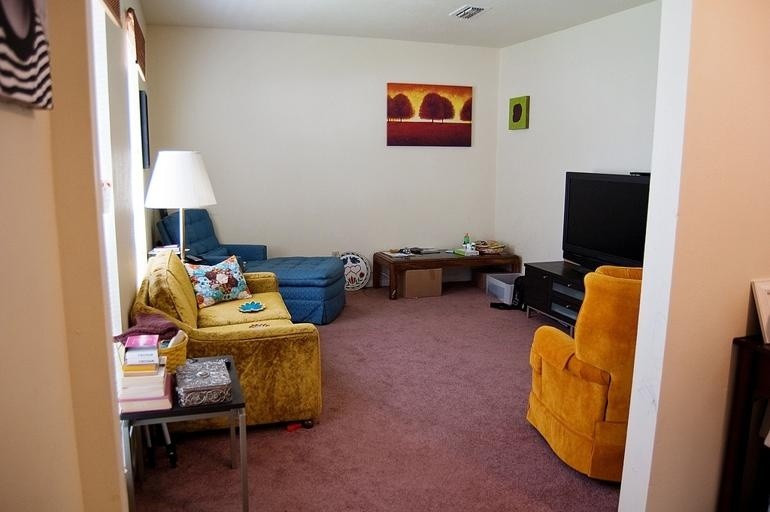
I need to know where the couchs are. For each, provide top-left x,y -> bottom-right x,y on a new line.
526,265 -> 643,484
156,209 -> 346,325
130,254 -> 322,428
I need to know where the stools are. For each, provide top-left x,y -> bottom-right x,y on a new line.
122,355 -> 249,511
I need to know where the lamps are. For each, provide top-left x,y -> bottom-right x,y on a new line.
449,6 -> 493,23
144,151 -> 217,264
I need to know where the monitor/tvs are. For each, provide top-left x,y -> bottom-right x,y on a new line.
561,170 -> 650,273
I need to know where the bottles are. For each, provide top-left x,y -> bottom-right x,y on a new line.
463,232 -> 469,251
470,242 -> 476,251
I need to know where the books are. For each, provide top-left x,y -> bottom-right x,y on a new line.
118,354 -> 172,414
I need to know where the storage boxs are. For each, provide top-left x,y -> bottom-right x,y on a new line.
473,272 -> 486,289
396,267 -> 442,299
486,273 -> 525,306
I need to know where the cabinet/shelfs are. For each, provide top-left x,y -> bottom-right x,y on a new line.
524,261 -> 596,339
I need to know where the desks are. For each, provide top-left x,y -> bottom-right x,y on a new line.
716,335 -> 770,512
372,247 -> 523,302
386,83 -> 474,148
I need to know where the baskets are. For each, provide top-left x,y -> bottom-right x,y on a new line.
158,329 -> 188,374
479,244 -> 504,254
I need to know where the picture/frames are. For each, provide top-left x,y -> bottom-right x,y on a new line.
508,96 -> 529,129
753,280 -> 770,345
